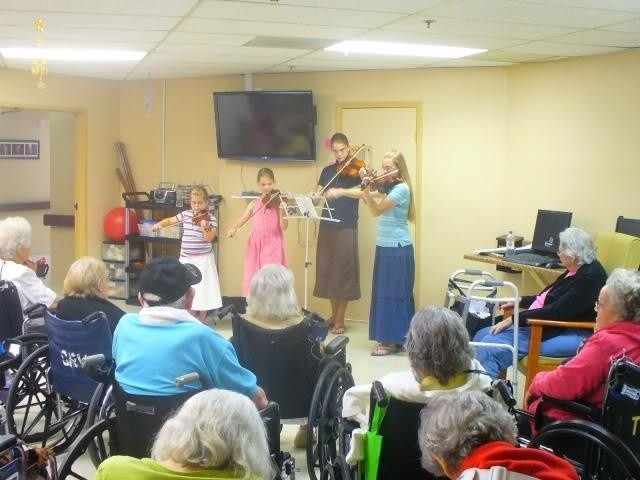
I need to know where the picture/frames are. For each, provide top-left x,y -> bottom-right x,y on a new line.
0,139 -> 40,159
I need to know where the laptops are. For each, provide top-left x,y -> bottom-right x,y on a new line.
502,208 -> 573,267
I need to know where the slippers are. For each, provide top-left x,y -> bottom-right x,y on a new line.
371,345 -> 392,356
331,321 -> 346,334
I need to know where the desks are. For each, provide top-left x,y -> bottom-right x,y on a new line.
463,240 -> 567,297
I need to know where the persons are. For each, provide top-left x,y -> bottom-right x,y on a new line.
358,150 -> 415,356
311,134 -> 369,335
418,389 -> 579,480
343,307 -> 492,428
473,228 -> 609,379
57,256 -> 129,401
0,217 -> 60,409
227,168 -> 288,298
518,268 -> 640,436
152,186 -> 223,321
95,257 -> 317,479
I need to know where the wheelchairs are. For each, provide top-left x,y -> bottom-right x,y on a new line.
321,380 -> 516,480
516,358 -> 640,480
214,304 -> 356,456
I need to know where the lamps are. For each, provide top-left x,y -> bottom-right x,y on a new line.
31,18 -> 48,90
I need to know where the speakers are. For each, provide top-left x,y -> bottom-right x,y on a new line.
496,233 -> 524,273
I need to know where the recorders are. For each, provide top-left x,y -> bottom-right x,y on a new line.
149,181 -> 176,204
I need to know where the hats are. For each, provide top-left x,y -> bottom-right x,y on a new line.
138,256 -> 202,307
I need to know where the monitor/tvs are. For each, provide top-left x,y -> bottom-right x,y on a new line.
213,90 -> 317,162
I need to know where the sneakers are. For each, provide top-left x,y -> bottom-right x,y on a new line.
293,430 -> 317,448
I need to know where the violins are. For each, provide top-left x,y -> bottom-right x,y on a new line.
192,210 -> 213,231
262,189 -> 294,215
337,155 -> 366,177
361,173 -> 400,193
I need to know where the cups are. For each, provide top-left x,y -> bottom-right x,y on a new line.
154,189 -> 166,203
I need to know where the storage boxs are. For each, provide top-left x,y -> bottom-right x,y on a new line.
137,223 -> 158,237
157,224 -> 183,239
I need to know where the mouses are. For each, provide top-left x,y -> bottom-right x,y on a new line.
545,263 -> 560,268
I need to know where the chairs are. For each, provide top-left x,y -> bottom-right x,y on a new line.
615,216 -> 640,237
499,229 -> 640,412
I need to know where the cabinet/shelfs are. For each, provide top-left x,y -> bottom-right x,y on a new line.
102,241 -> 145,300
123,191 -> 223,306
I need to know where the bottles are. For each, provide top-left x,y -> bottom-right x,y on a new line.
506,231 -> 515,257
176,191 -> 183,207
